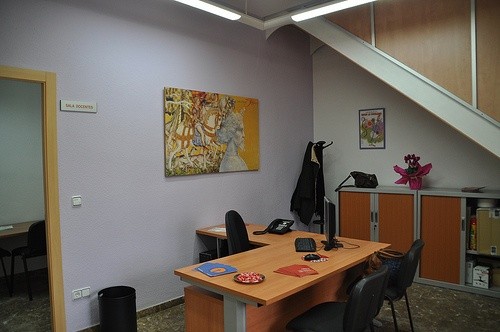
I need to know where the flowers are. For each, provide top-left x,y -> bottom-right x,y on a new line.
404,153 -> 420,173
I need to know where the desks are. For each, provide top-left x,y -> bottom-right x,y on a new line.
0,220 -> 38,238
175,223 -> 392,332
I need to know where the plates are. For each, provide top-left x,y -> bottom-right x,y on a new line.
233,272 -> 265,284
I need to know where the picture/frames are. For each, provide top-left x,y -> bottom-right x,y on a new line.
359,108 -> 385,149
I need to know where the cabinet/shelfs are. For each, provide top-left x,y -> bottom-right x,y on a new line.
336,186 -> 418,283
416,187 -> 500,297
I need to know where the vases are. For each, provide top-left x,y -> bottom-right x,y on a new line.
409,179 -> 422,189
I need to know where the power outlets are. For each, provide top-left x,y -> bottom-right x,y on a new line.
72,289 -> 82,300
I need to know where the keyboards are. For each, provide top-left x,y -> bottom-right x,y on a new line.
294,238 -> 316,252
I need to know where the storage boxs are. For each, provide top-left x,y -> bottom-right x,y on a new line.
472,265 -> 500,289
476,207 -> 500,256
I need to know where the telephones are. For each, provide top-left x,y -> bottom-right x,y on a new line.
268,219 -> 294,235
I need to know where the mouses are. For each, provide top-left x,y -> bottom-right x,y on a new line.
304,254 -> 320,261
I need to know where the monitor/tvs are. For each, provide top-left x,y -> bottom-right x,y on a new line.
321,196 -> 343,251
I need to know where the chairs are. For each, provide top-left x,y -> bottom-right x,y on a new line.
225,210 -> 250,255
0,248 -> 11,283
286,265 -> 389,332
10,220 -> 49,301
345,239 -> 425,332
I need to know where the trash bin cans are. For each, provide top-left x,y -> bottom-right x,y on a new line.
98,286 -> 137,332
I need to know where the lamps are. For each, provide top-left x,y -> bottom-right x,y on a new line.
290,0 -> 377,22
175,0 -> 241,21
